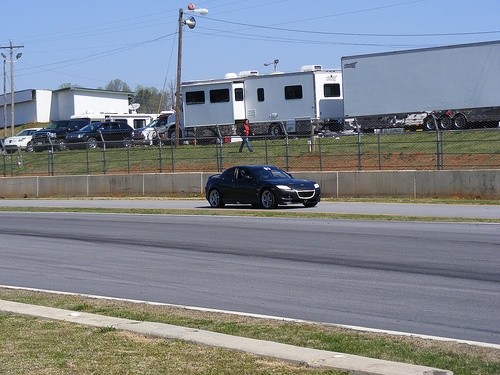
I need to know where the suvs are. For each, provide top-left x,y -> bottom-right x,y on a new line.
4,127 -> 44,154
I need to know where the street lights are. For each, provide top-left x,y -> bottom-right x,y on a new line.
1,52 -> 22,136
175,8 -> 208,146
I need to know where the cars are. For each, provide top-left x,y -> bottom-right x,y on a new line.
26,128 -> 66,152
65,122 -> 134,149
205,165 -> 321,209
132,113 -> 184,146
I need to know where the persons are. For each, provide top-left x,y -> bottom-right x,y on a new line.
238,118 -> 253,152
